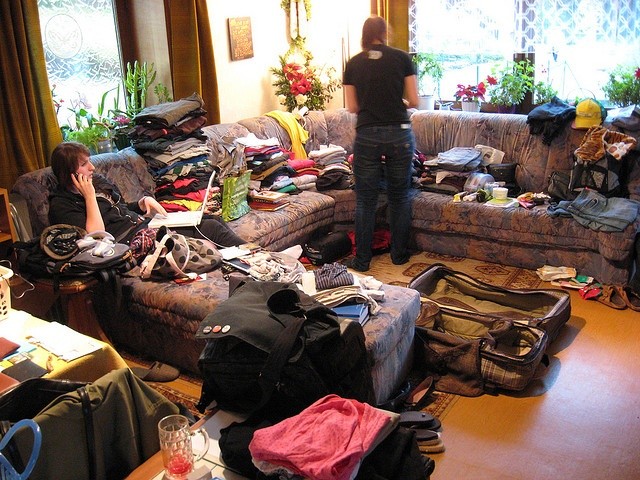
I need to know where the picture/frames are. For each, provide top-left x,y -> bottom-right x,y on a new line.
228,17 -> 253,62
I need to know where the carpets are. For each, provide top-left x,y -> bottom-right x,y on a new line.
115,245 -> 547,426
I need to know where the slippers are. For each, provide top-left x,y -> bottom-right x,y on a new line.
129,360 -> 181,382
612,283 -> 640,309
591,283 -> 627,310
399,411 -> 442,431
403,424 -> 444,454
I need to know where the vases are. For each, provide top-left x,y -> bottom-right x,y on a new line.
114,134 -> 132,149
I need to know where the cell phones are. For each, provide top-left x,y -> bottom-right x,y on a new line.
69,172 -> 83,184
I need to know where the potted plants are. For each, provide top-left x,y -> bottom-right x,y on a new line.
438,99 -> 453,110
488,59 -> 532,114
461,102 -> 481,113
407,53 -> 442,110
75,88 -> 112,153
68,128 -> 99,156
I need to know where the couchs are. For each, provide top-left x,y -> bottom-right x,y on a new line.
237,107 -> 417,239
119,122 -> 336,269
12,153 -> 421,408
385,110 -> 640,290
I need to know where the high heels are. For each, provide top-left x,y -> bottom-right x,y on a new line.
404,376 -> 434,409
377,389 -> 409,411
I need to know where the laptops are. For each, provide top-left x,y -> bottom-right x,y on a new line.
148,171 -> 217,229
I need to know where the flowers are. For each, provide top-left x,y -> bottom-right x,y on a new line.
602,66 -> 640,108
110,114 -> 134,132
453,76 -> 497,102
269,37 -> 343,112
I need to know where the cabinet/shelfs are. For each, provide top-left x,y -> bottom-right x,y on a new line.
0,188 -> 19,281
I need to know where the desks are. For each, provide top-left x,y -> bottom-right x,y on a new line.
23,269 -> 115,349
0,307 -> 127,405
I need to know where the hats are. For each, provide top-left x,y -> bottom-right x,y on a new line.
572,100 -> 602,128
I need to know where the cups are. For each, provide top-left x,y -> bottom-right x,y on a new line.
493,187 -> 508,203
158,415 -> 208,479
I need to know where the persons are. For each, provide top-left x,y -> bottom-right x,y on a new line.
48,143 -> 248,250
342,17 -> 420,273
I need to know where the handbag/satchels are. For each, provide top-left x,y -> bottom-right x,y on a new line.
0,380 -> 94,479
486,162 -> 518,193
9,202 -> 29,244
549,170 -> 569,198
304,230 -> 353,265
138,226 -> 222,281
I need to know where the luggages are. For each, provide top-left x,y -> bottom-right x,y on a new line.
400,264 -> 572,391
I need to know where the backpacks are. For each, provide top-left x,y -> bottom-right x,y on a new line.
199,278 -> 339,421
23,235 -> 131,277
